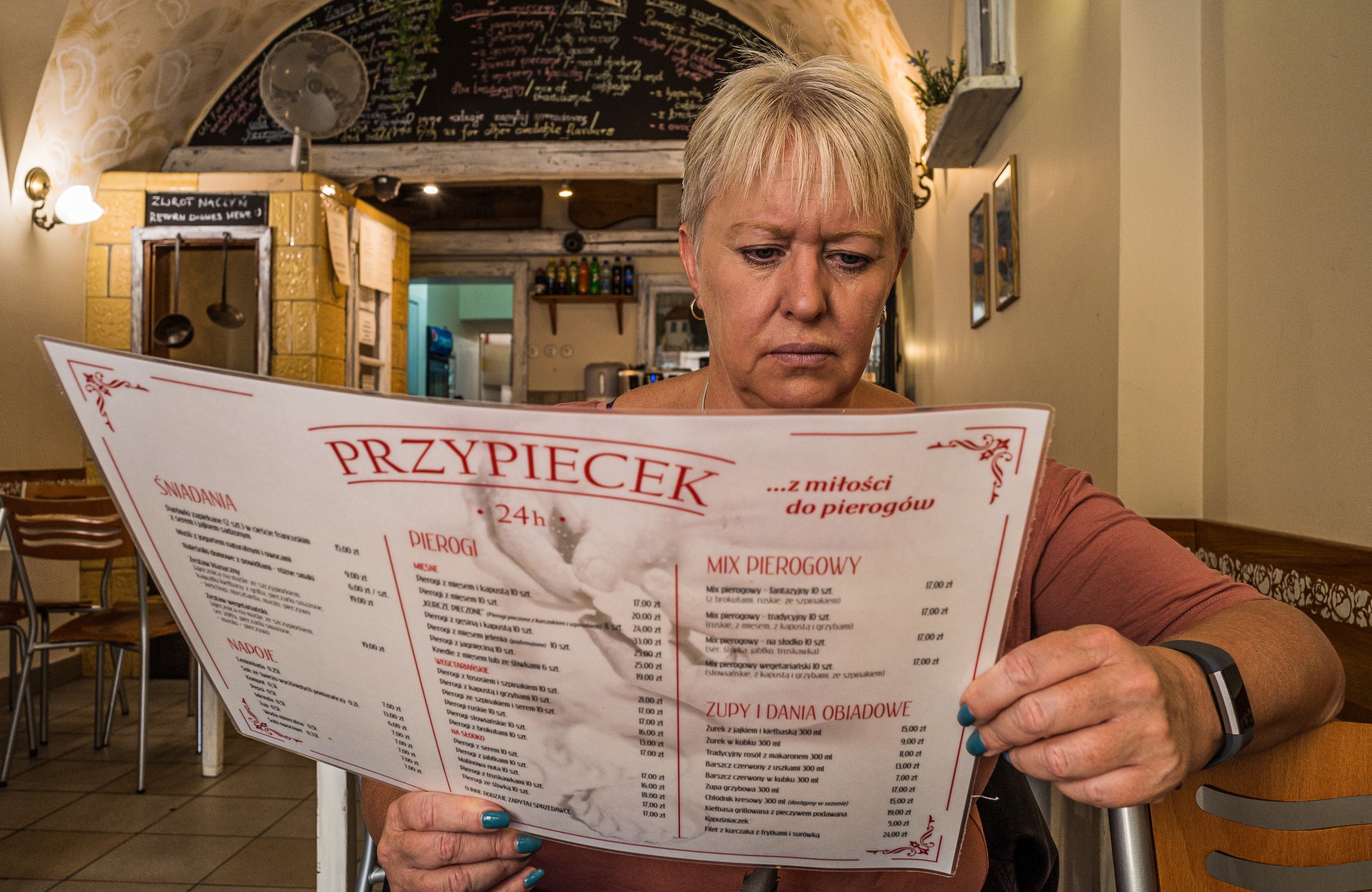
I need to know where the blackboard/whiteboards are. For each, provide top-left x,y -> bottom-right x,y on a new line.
179,0 -> 803,146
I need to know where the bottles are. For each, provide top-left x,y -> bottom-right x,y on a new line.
535,255 -> 634,295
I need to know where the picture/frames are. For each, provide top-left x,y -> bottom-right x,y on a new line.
992,153 -> 1021,312
968,193 -> 991,330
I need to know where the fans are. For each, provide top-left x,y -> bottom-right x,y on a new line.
258,29 -> 370,172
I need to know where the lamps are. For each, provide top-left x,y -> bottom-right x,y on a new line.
912,142 -> 934,210
25,167 -> 104,231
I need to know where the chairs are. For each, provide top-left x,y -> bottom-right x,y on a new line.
0,474 -> 205,796
1105,722 -> 1372,892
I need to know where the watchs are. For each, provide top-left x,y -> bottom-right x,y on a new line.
1154,640 -> 1255,790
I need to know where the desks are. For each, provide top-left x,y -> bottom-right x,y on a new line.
0,506 -> 227,779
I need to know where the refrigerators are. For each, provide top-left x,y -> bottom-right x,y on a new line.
427,325 -> 453,397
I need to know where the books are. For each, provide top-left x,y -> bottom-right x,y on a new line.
36,334 -> 1057,877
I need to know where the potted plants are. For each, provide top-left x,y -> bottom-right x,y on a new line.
906,40 -> 967,145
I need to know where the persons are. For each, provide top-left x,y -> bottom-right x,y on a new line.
378,32 -> 1349,890
